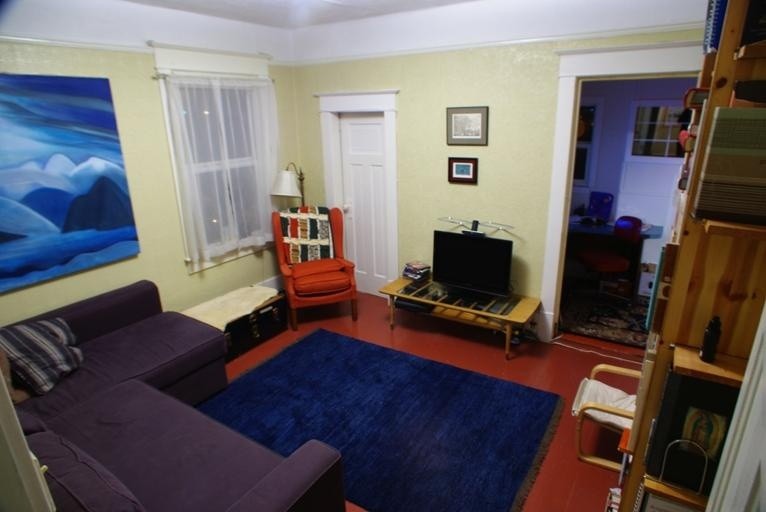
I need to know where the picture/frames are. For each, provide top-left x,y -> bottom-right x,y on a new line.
0,73 -> 141,293
445,105 -> 489,146
448,156 -> 478,184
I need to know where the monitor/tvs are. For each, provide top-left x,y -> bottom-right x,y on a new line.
432,229 -> 513,304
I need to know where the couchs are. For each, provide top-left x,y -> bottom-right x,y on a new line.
0,280 -> 346,511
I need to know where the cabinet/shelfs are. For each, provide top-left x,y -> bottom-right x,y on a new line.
616,0 -> 766,512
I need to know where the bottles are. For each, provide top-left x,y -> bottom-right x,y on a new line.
699,315 -> 722,363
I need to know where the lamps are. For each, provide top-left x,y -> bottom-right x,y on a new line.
269,161 -> 305,206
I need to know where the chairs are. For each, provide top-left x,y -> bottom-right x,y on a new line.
572,362 -> 642,473
272,206 -> 359,331
568,216 -> 642,323
586,191 -> 614,221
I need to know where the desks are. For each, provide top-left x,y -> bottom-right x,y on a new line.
379,275 -> 541,358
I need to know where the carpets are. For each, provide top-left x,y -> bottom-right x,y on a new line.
563,298 -> 649,349
190,329 -> 565,512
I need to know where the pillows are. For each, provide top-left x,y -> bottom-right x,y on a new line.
0,317 -> 86,392
